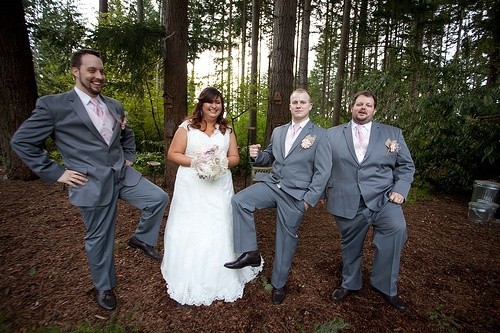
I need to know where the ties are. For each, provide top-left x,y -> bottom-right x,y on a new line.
90,98 -> 103,118
355,125 -> 364,141
292,124 -> 301,139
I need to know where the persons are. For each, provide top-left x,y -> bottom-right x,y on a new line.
10,50 -> 167,311
224,88 -> 332,304
167,87 -> 240,271
328,91 -> 415,312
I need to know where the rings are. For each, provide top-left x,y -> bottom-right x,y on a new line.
398,200 -> 401,202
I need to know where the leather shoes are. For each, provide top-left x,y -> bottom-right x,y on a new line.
224,251 -> 261,269
332,286 -> 358,302
372,286 -> 406,311
95,289 -> 116,311
127,235 -> 163,260
272,285 -> 286,304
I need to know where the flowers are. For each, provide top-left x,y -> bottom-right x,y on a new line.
195,146 -> 229,180
299,135 -> 315,151
121,110 -> 128,130
385,139 -> 401,156
